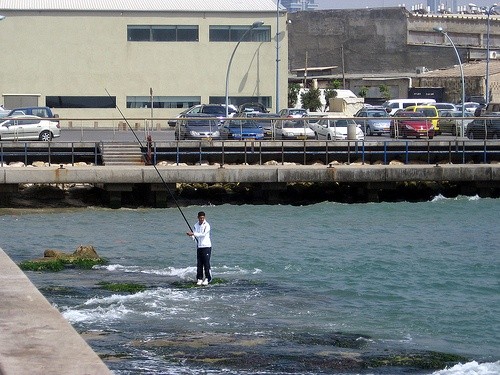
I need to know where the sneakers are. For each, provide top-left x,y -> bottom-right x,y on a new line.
204,276 -> 209,289
197,279 -> 199,287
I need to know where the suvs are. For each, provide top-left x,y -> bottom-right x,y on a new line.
1,105 -> 60,125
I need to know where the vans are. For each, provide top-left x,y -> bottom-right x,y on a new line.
405,106 -> 439,135
383,98 -> 436,117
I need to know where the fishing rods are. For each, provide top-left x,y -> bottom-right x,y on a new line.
103,87 -> 200,242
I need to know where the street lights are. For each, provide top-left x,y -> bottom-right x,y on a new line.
468,2 -> 498,103
224,20 -> 265,119
432,26 -> 465,137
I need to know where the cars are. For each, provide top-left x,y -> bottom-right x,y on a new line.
174,112 -> 226,140
389,111 -> 435,139
0,115 -> 62,142
274,115 -> 315,140
357,111 -> 392,135
167,102 -> 308,134
466,116 -> 500,139
309,115 -> 364,140
354,102 -> 500,116
223,113 -> 265,140
439,111 -> 476,136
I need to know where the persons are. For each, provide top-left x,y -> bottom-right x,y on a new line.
187,212 -> 213,285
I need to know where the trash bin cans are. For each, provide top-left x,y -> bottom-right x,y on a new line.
347,124 -> 357,140
